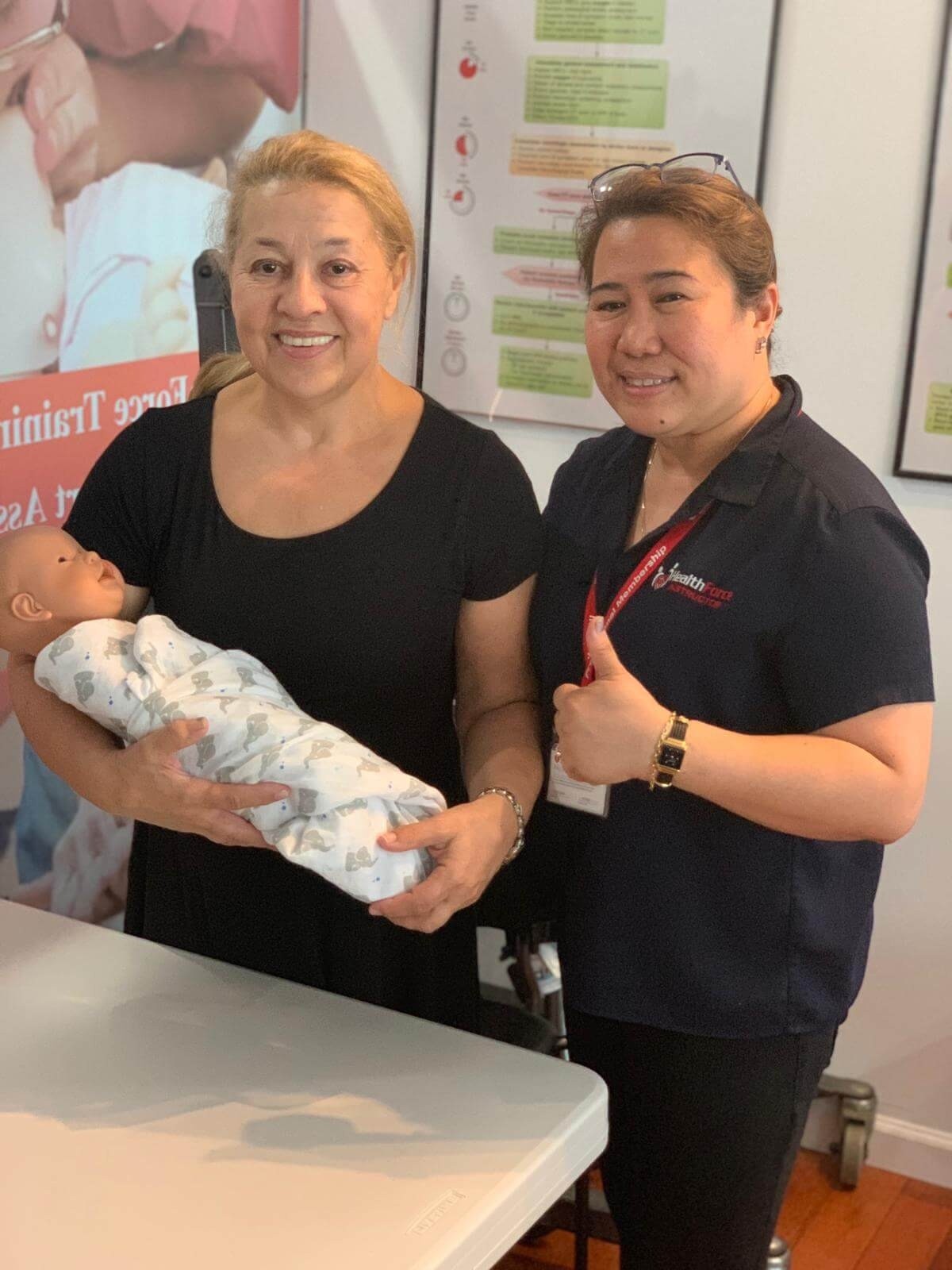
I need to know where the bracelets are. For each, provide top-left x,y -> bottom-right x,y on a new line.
650,710 -> 676,792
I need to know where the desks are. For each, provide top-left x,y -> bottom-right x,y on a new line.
0,895 -> 609,1270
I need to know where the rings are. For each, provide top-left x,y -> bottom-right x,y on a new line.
555,752 -> 563,762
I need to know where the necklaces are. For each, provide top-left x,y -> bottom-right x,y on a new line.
641,388 -> 773,536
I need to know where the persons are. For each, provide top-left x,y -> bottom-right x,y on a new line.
0,129 -> 541,1037
527,168 -> 937,1269
0,0 -> 298,386
0,523 -> 447,905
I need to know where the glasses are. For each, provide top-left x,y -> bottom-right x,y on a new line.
586,152 -> 744,217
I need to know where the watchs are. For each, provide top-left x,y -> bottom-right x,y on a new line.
655,715 -> 688,789
475,787 -> 524,868
